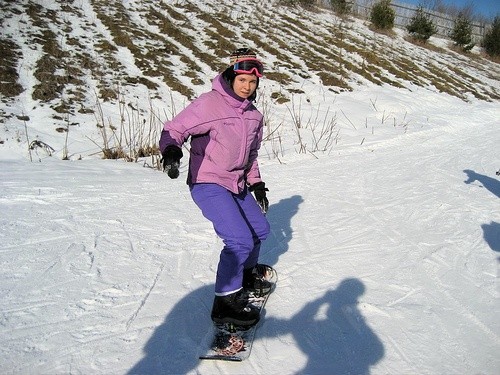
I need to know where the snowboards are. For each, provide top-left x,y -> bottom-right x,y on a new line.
197,263 -> 278,362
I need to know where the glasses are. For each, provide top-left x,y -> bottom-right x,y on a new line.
233,58 -> 264,77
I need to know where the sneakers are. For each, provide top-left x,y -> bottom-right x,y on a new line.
242,268 -> 274,297
209,293 -> 261,326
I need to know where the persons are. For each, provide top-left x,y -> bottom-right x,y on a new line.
158,58 -> 277,325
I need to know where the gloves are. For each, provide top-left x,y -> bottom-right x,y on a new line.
160,145 -> 184,180
248,182 -> 269,217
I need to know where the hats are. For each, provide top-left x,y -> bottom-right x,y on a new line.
230,47 -> 256,66
